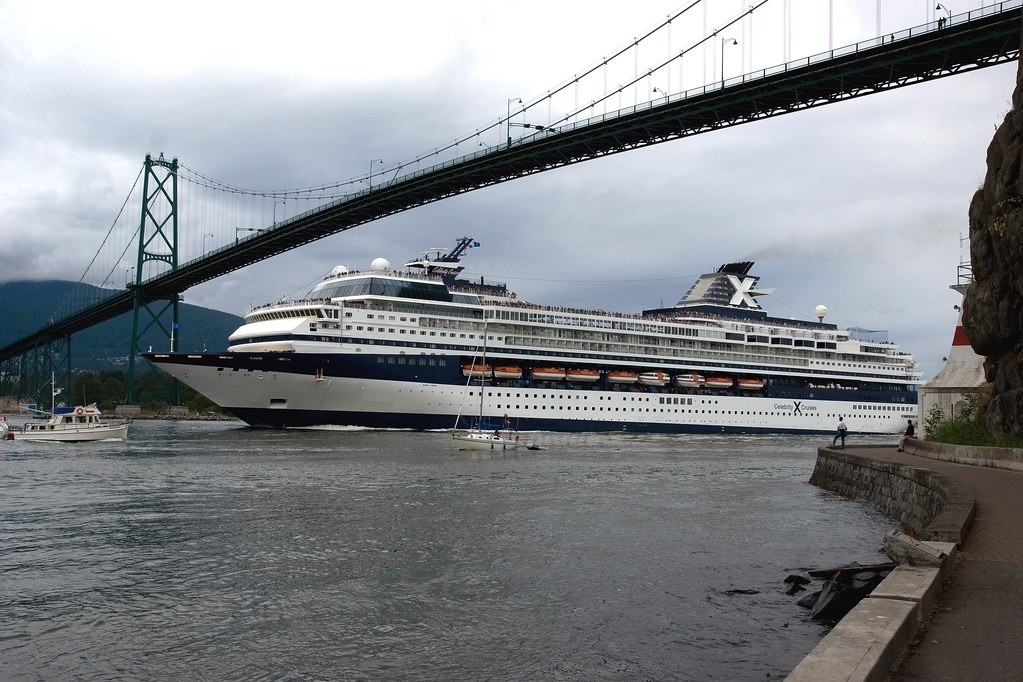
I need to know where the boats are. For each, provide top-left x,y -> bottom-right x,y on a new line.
531,367 -> 566,380
672,373 -> 706,388
637,372 -> 671,386
494,366 -> 523,379
607,371 -> 639,384
736,379 -> 764,390
565,369 -> 600,382
0,370 -> 135,442
463,364 -> 493,378
704,377 -> 733,389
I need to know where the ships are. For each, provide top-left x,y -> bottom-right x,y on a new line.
138,233 -> 929,434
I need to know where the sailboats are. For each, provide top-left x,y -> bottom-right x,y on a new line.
451,315 -> 548,451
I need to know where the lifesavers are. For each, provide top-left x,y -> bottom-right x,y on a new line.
77,408 -> 83,414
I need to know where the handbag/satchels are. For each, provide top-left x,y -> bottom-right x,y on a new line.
844,431 -> 846,437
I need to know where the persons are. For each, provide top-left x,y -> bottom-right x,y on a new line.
896,420 -> 914,453
831,417 -> 847,449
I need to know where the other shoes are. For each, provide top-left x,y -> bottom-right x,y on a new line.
840,447 -> 844,449
830,446 -> 834,449
896,448 -> 904,453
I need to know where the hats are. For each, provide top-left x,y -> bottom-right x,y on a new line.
908,420 -> 912,422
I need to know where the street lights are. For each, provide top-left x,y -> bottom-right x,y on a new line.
507,97 -> 523,149
106,281 -> 115,300
936,3 -> 952,27
202,233 -> 214,259
720,37 -> 738,88
131,266 -> 135,285
479,142 -> 492,154
369,158 -> 384,194
273,200 -> 285,230
653,87 -> 667,105
125,270 -> 129,290
